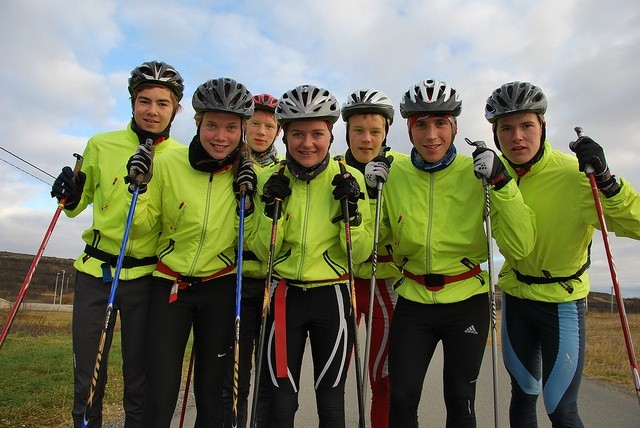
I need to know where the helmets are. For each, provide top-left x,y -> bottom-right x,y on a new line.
245,93 -> 280,155
127,60 -> 184,141
192,77 -> 255,162
274,84 -> 340,173
484,80 -> 546,168
342,88 -> 394,167
399,77 -> 461,151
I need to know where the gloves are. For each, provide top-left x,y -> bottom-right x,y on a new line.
570,137 -> 615,191
473,147 -> 509,187
365,154 -> 394,196
331,173 -> 360,223
50,166 -> 86,206
123,144 -> 156,189
262,171 -> 293,215
232,162 -> 258,203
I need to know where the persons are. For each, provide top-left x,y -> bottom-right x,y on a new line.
124,77 -> 255,428
234,95 -> 282,427
254,85 -> 369,428
341,91 -> 412,428
364,79 -> 538,427
484,81 -> 637,426
53,61 -> 188,427
